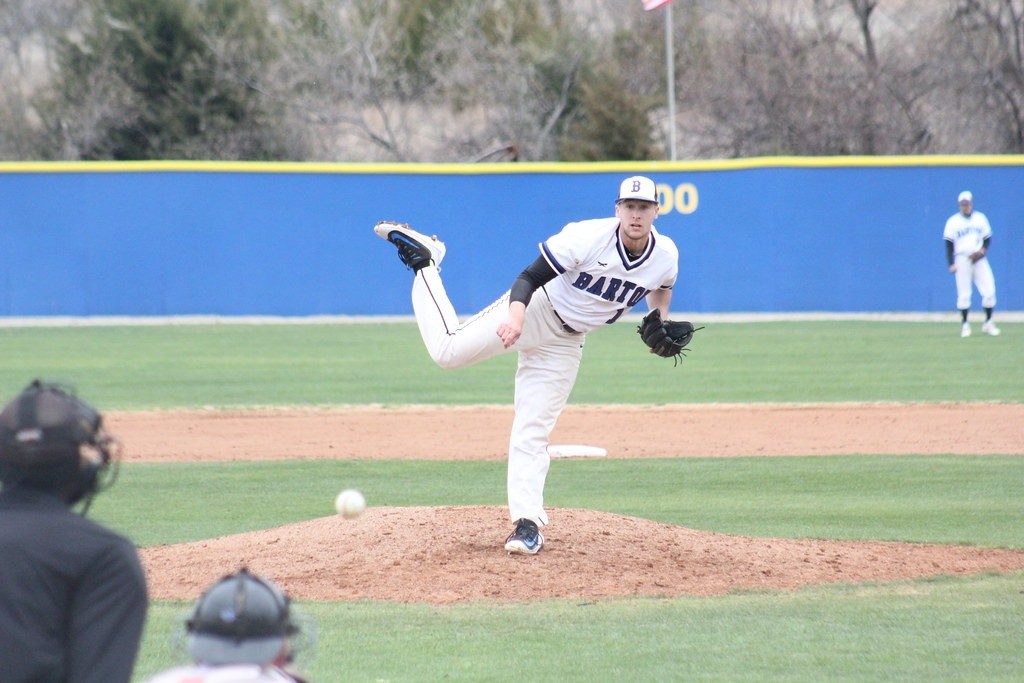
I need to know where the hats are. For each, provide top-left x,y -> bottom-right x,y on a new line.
958,190 -> 973,205
614,176 -> 658,203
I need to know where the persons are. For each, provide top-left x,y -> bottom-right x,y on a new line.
0,385 -> 149,683
942,190 -> 1002,338
372,176 -> 693,555
151,570 -> 309,683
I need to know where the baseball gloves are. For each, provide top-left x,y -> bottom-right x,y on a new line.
638,307 -> 695,358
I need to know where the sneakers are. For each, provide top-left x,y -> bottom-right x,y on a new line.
961,322 -> 971,337
981,319 -> 1001,336
374,220 -> 446,273
505,518 -> 544,554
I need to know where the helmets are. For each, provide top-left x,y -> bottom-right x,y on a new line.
185,566 -> 311,663
1,378 -> 124,515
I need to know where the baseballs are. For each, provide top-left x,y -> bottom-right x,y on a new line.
335,488 -> 365,518
